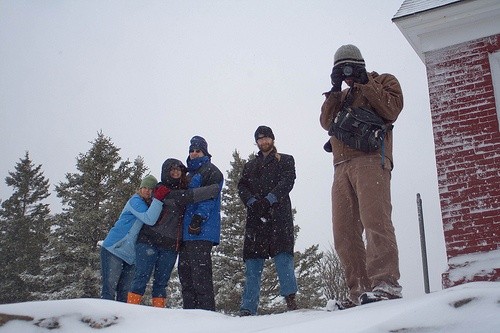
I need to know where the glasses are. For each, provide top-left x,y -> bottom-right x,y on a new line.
190,149 -> 201,153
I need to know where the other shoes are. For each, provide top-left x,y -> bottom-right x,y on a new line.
360,290 -> 401,304
333,299 -> 357,309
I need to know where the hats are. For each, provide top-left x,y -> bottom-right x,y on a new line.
189,136 -> 207,154
139,175 -> 157,189
254,126 -> 275,142
334,45 -> 365,67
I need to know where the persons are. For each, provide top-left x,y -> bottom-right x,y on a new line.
128,158 -> 219,308
320,44 -> 404,311
100,176 -> 172,300
239,126 -> 297,315
175,135 -> 224,310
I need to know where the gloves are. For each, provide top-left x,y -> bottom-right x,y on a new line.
188,215 -> 203,235
346,64 -> 369,84
250,197 -> 270,218
153,186 -> 171,200
331,65 -> 345,91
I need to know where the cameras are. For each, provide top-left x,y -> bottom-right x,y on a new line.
339,63 -> 355,77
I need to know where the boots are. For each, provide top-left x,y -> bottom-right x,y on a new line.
152,298 -> 164,308
240,312 -> 250,317
285,294 -> 297,309
127,292 -> 142,305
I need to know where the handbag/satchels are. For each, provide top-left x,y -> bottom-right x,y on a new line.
330,107 -> 387,152
